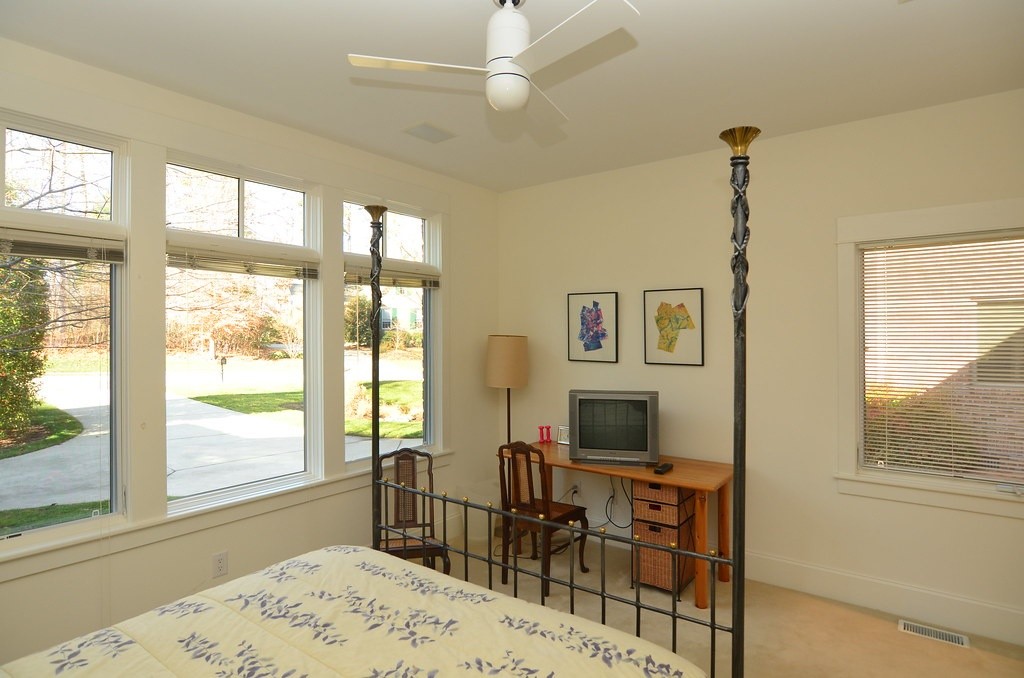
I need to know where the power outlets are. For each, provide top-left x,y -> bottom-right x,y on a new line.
608,487 -> 618,506
571,480 -> 582,497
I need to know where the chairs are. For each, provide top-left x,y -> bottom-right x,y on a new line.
498,441 -> 590,597
379,447 -> 452,576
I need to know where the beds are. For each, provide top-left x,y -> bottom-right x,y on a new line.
0,125 -> 760,678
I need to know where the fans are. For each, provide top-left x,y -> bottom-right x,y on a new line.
347,0 -> 640,129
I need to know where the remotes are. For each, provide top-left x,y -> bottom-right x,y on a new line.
654,463 -> 673,474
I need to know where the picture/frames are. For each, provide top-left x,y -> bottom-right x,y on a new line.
566,292 -> 618,363
644,287 -> 705,367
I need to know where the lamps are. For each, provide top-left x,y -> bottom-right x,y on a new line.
484,335 -> 529,538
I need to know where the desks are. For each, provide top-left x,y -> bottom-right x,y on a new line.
495,440 -> 733,609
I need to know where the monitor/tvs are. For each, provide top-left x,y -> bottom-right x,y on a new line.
568,389 -> 659,466
